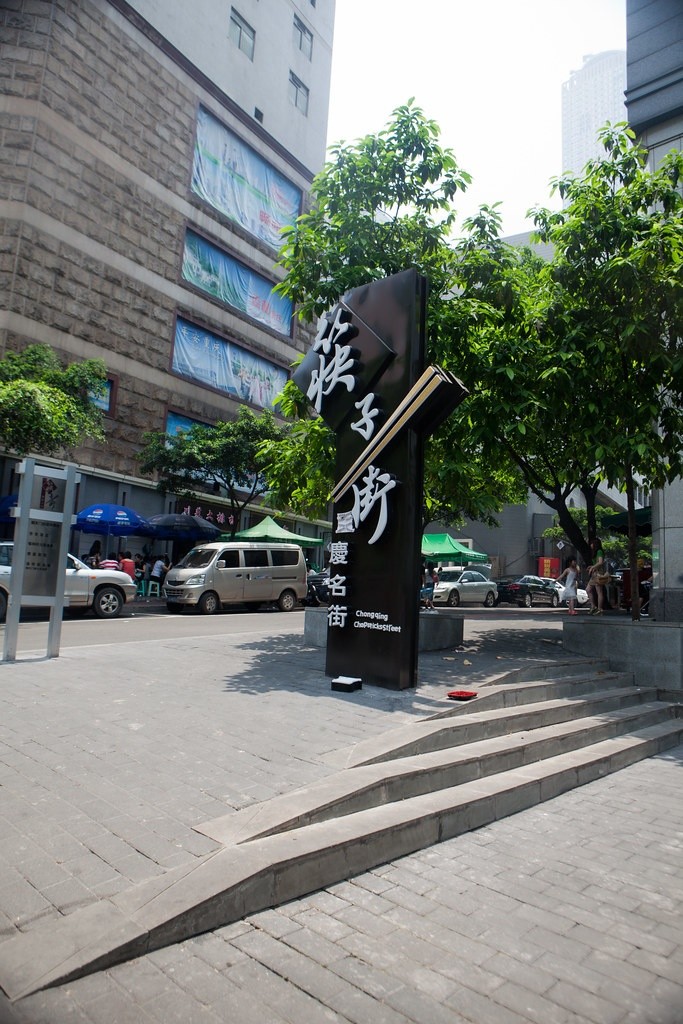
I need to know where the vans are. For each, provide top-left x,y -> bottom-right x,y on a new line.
161,542 -> 309,614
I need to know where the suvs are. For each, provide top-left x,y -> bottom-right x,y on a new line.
0,542 -> 138,619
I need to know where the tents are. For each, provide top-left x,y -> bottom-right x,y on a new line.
421,533 -> 489,590
218,516 -> 323,577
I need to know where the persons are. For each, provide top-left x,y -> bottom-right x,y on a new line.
585,537 -> 605,616
306,564 -> 316,576
420,556 -> 440,610
82,552 -> 173,584
638,556 -> 653,618
553,555 -> 581,616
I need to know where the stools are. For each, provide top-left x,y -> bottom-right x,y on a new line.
136,580 -> 146,596
147,581 -> 160,597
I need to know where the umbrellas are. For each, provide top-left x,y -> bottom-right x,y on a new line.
71,505 -> 156,596
146,515 -> 222,597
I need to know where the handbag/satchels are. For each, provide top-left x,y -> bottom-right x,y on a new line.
432,575 -> 438,581
593,572 -> 611,585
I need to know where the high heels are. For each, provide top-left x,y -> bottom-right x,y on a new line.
587,606 -> 604,616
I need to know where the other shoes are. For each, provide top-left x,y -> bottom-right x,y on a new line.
425,605 -> 428,609
568,609 -> 578,615
430,605 -> 434,609
640,611 -> 649,617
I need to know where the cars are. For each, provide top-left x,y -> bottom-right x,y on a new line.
540,577 -> 589,606
489,574 -> 561,608
418,570 -> 499,607
306,565 -> 331,606
